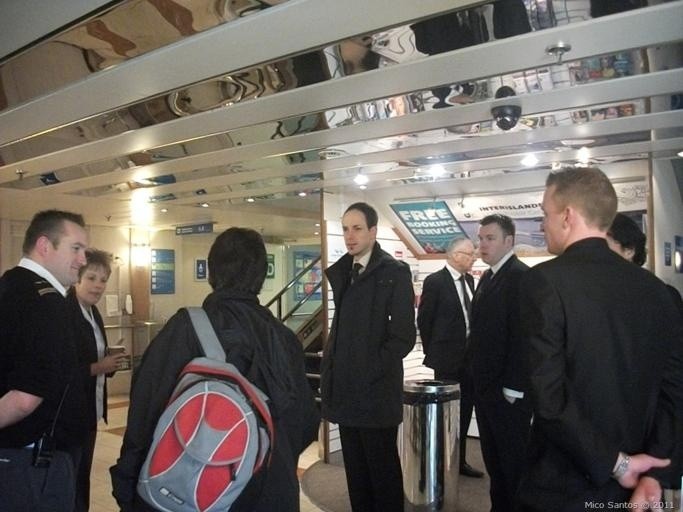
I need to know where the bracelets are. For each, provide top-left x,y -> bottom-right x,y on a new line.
611,456 -> 630,479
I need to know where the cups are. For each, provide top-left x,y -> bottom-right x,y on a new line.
105,345 -> 126,372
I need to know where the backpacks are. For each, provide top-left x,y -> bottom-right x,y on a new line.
133,304 -> 275,512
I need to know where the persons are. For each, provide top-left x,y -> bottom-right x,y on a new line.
111,228 -> 325,512
607,213 -> 649,267
518,166 -> 682,510
0,209 -> 89,512
325,202 -> 417,512
417,237 -> 484,478
470,214 -> 534,512
69,249 -> 133,511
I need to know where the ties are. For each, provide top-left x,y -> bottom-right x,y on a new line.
476,269 -> 492,305
460,274 -> 473,322
348,263 -> 363,280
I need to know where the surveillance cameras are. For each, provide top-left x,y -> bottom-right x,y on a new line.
496,86 -> 515,98
492,105 -> 521,130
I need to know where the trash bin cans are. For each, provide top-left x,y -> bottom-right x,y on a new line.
132,319 -> 165,358
402,379 -> 463,510
